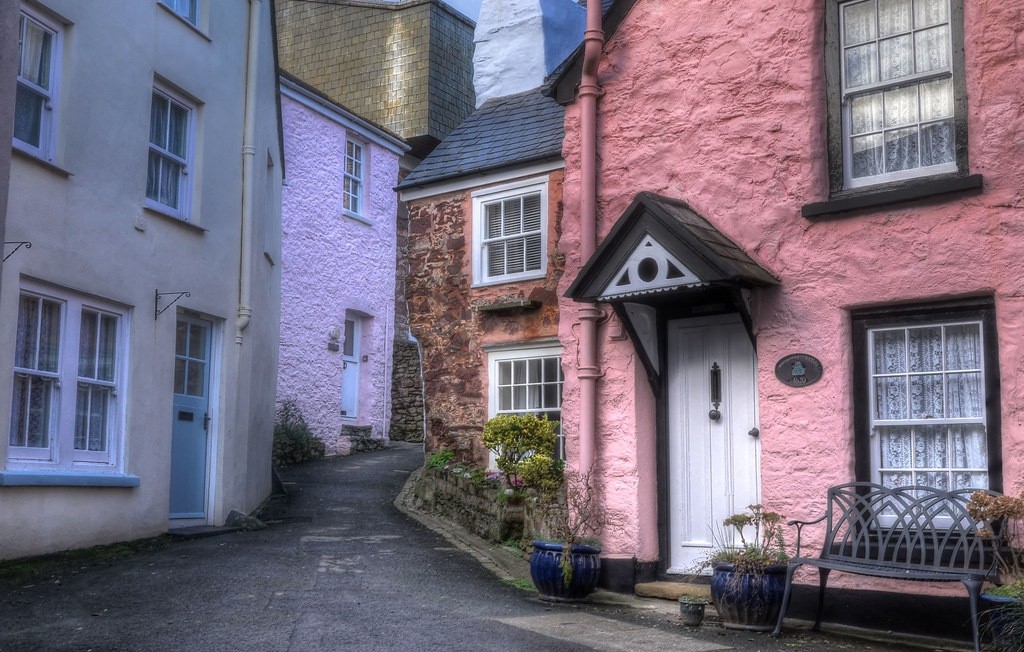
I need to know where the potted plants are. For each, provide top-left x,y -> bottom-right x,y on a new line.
677,587 -> 706,627
969,490 -> 1024,652
681,504 -> 796,634
530,477 -> 608,599
481,412 -> 565,507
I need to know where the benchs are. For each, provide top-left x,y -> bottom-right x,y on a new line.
772,481 -> 1002,652
